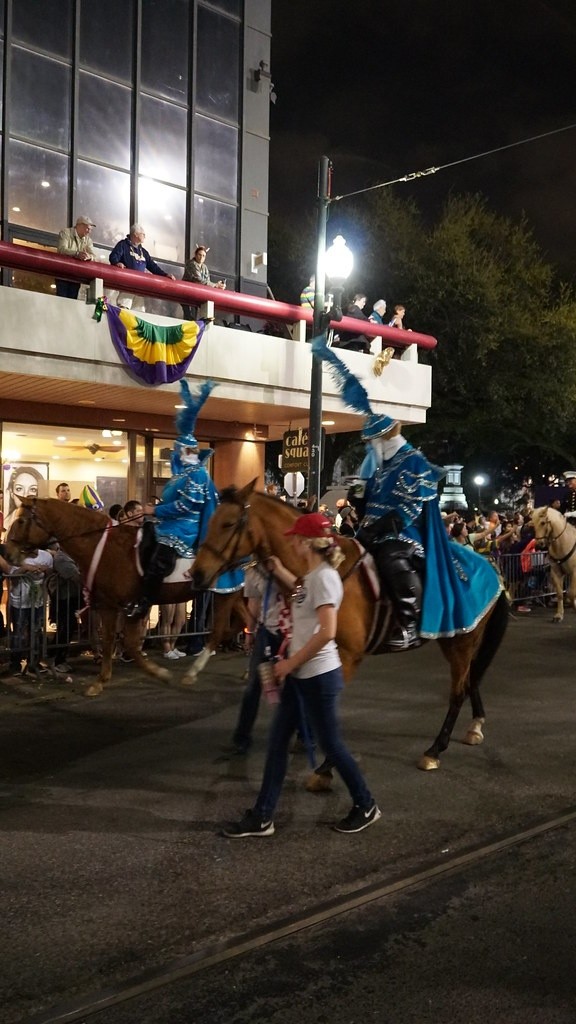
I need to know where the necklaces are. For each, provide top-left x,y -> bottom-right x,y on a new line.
130,246 -> 143,261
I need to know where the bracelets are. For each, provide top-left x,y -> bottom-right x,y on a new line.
244,628 -> 254,634
76,252 -> 79,256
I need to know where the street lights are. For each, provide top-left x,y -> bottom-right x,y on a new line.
308,227 -> 356,513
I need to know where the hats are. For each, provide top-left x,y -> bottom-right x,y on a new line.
173,380 -> 214,447
311,336 -> 399,439
563,471 -> 576,482
283,514 -> 335,537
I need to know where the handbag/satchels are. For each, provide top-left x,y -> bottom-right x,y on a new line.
247,625 -> 288,688
269,655 -> 285,687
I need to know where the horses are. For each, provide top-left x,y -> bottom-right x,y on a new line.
189,476 -> 509,792
527,506 -> 576,624
4,493 -> 260,698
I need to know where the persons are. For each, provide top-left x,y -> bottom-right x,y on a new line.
216,560 -> 289,756
221,513 -> 382,838
3,467 -> 45,529
300,275 -> 315,307
352,413 -> 448,647
179,247 -> 226,321
127,434 -> 220,617
391,306 -> 412,359
0,471 -> 576,673
369,299 -> 387,325
340,293 -> 377,353
107,224 -> 175,312
55,215 -> 96,299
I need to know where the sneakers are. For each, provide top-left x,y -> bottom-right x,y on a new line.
518,606 -> 531,611
334,798 -> 382,833
55,662 -> 72,672
192,647 -> 216,656
164,651 -> 179,659
222,809 -> 274,837
173,648 -> 187,656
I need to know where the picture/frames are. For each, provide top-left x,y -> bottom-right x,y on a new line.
2,460 -> 49,533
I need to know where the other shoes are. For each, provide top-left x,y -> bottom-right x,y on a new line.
298,734 -> 317,751
215,746 -> 246,759
28,664 -> 48,672
121,654 -> 134,662
12,667 -> 22,675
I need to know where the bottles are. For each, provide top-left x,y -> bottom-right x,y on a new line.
222,278 -> 226,283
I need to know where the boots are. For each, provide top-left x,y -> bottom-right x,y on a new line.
384,570 -> 423,645
126,575 -> 160,618
